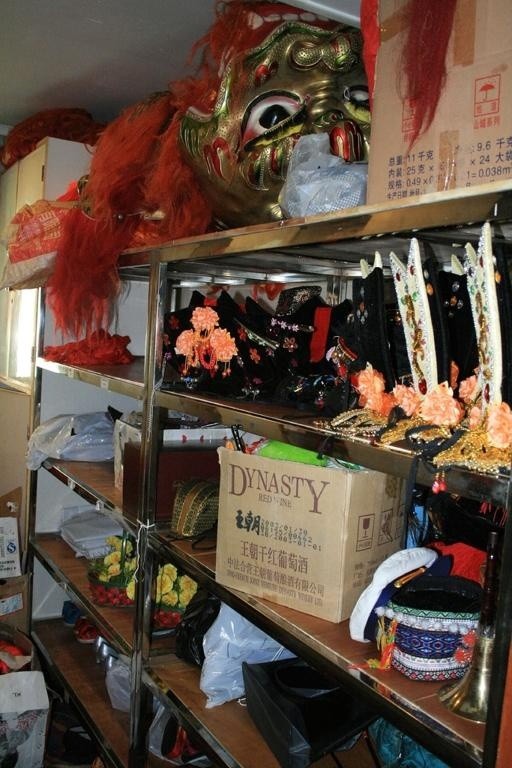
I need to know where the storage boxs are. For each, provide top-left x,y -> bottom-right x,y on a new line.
212,441 -> 408,625
364,0 -> 511,205
0,486 -> 53,767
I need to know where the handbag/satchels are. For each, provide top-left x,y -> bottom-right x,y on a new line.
173,586 -> 298,709
241,655 -> 384,768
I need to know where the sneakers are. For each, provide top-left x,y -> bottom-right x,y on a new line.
61,599 -> 98,645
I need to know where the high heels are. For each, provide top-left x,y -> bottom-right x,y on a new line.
93,635 -> 119,673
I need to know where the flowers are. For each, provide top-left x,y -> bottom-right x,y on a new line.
87,530 -> 200,616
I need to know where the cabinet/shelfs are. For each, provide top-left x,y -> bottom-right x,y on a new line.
31,175 -> 511,767
0,136 -> 99,552
